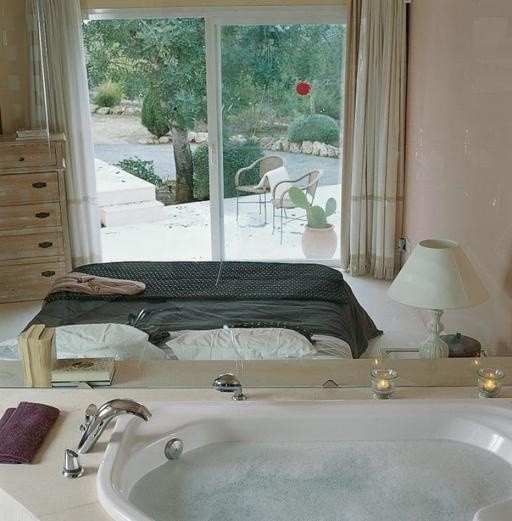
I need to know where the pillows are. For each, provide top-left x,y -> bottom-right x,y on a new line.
1,322 -> 166,366
166,328 -> 317,363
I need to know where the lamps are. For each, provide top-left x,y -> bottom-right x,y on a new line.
386,238 -> 489,358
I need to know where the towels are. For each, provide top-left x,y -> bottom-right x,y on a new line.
1,402 -> 59,464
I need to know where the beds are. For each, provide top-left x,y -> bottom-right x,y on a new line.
17,261 -> 355,363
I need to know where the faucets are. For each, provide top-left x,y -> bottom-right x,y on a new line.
79,399 -> 151,454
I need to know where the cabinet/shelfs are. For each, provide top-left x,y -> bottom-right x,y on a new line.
0,132 -> 76,305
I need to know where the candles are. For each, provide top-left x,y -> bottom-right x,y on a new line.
483,378 -> 497,391
376,379 -> 391,393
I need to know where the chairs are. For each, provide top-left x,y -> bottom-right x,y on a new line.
235,155 -> 284,226
272,169 -> 324,246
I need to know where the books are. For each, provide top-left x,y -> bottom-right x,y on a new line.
15,127 -> 52,140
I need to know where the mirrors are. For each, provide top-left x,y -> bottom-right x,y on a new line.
1,0 -> 512,386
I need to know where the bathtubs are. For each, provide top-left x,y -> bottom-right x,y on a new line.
97,398 -> 512,521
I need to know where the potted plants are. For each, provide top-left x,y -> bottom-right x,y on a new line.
289,186 -> 338,261
